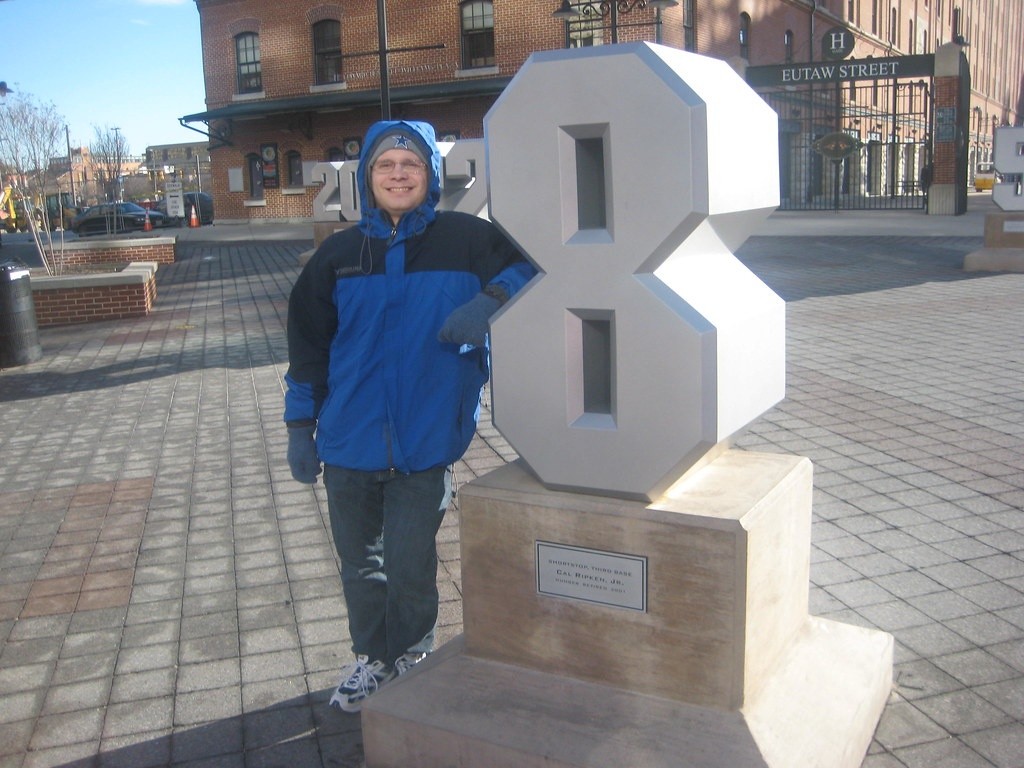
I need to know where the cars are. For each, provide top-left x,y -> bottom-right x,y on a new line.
153,192 -> 214,227
73,201 -> 165,236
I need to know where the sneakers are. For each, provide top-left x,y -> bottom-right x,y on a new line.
329,661 -> 399,713
394,652 -> 427,676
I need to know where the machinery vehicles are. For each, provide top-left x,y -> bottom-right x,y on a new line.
0,186 -> 86,233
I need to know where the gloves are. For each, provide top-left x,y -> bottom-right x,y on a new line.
437,292 -> 501,348
287,422 -> 323,483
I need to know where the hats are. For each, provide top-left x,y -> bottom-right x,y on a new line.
365,134 -> 430,180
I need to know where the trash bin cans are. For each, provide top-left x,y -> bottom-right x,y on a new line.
0,254 -> 44,365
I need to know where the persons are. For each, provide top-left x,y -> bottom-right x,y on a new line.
284,121 -> 538,713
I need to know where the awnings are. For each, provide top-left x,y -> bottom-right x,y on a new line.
178,75 -> 514,141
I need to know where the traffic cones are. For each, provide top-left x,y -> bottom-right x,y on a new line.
142,207 -> 153,232
188,203 -> 200,227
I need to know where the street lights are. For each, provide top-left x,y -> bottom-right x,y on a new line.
111,127 -> 124,201
142,150 -> 157,200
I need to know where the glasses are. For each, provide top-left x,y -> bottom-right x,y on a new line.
369,159 -> 429,174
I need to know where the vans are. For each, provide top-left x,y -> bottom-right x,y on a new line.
974,162 -> 996,192
